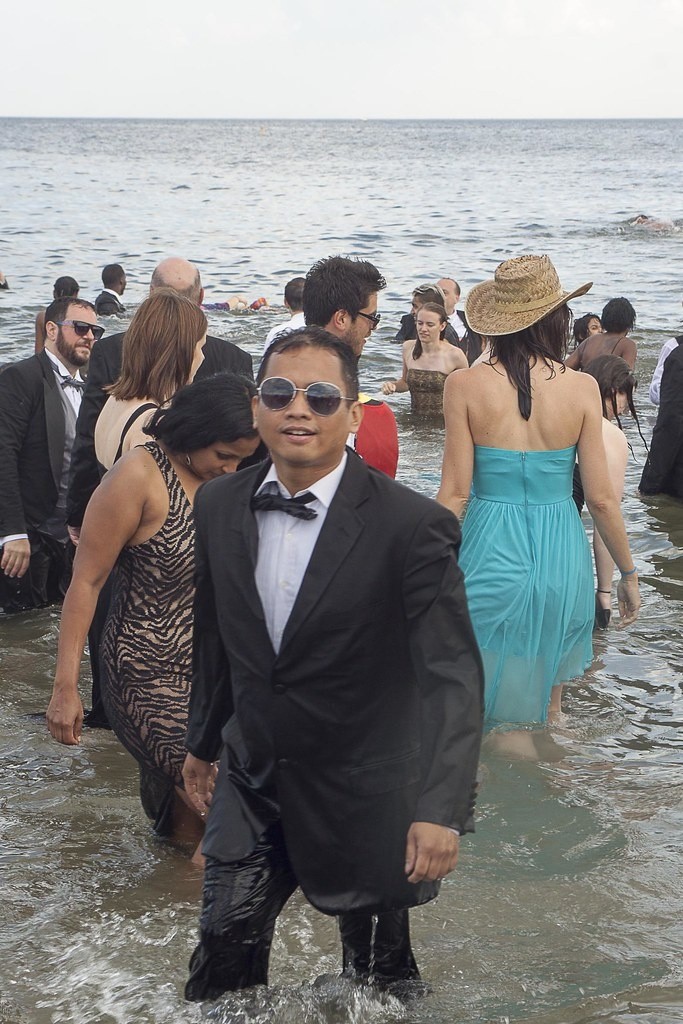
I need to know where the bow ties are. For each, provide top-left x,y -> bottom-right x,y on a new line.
250,492 -> 319,519
48,356 -> 85,391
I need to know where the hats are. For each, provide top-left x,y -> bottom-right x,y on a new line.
464,255 -> 593,336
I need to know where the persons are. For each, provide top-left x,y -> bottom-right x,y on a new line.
95,264 -> 127,315
382,302 -> 468,417
34,276 -> 80,355
46,371 -> 261,836
0,296 -> 105,613
94,293 -> 207,481
435,255 -> 641,727
262,277 -> 306,361
203,295 -> 247,311
66,257 -> 254,547
180,328 -> 486,1002
564,297 -> 637,370
303,256 -> 387,450
573,314 -> 603,346
573,354 -> 637,624
637,334 -> 683,499
398,278 -> 482,368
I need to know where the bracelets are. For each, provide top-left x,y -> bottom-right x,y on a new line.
621,566 -> 637,576
598,589 -> 611,593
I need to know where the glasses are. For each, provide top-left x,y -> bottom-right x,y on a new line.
257,377 -> 354,417
54,321 -> 105,340
333,307 -> 380,329
